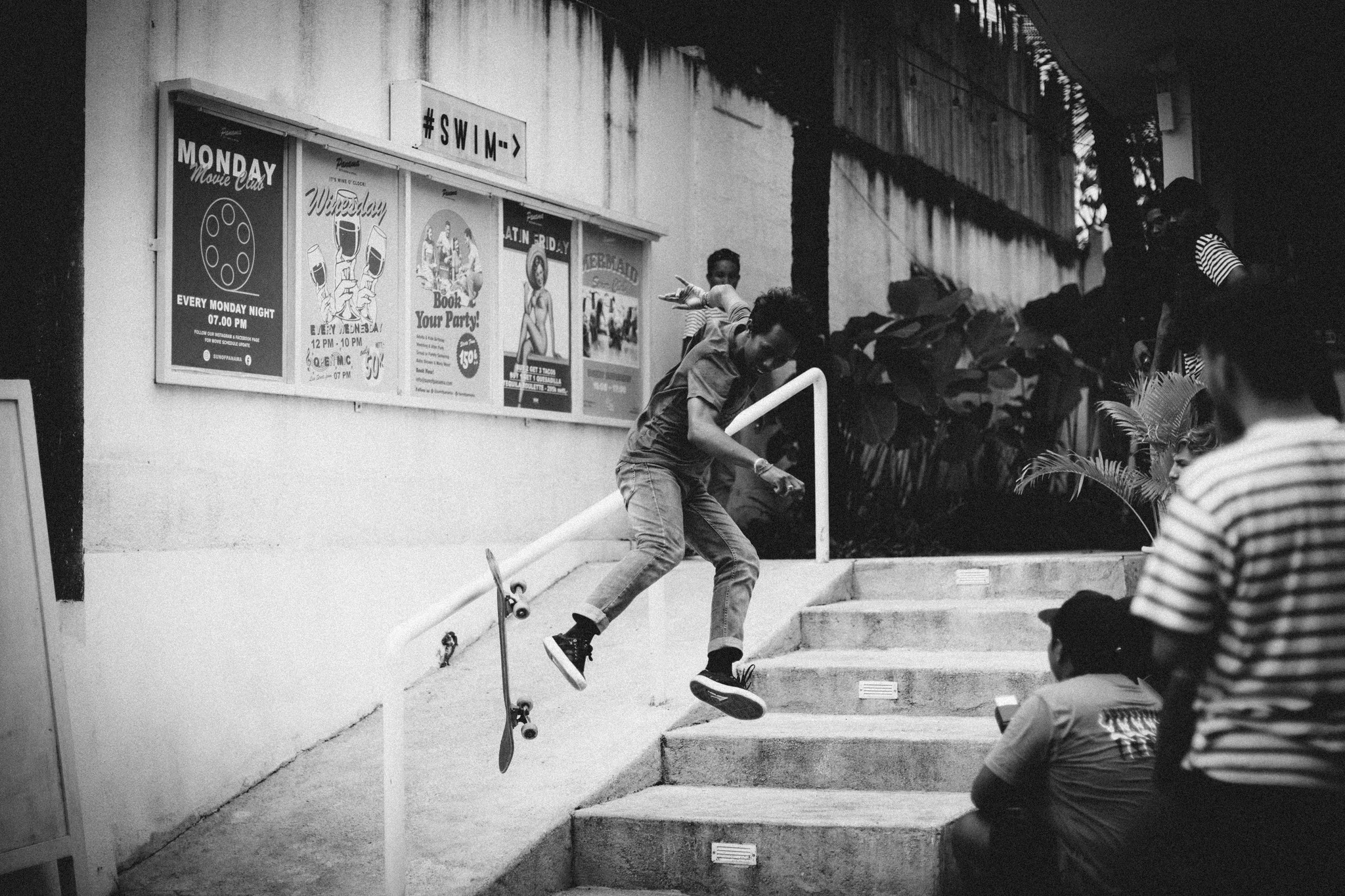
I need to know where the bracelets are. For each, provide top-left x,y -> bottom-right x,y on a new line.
754,458 -> 774,476
702,292 -> 714,309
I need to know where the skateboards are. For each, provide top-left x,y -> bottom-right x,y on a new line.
483,548 -> 539,776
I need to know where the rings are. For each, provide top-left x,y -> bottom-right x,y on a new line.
787,486 -> 792,490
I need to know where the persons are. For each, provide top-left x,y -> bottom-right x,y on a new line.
1169,426 -> 1223,486
542,274 -> 820,720
416,225 -> 438,289
435,221 -> 453,276
682,249 -> 740,509
451,237 -> 477,298
459,227 -> 483,307
513,243 -> 562,408
1129,292 -> 1345,895
939,591 -> 1163,896
1124,196 -> 1168,369
1150,176 -> 1247,384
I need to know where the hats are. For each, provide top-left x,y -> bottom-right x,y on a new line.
1039,588 -> 1131,657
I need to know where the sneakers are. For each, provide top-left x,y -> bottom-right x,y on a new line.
689,663 -> 766,721
543,632 -> 593,690
